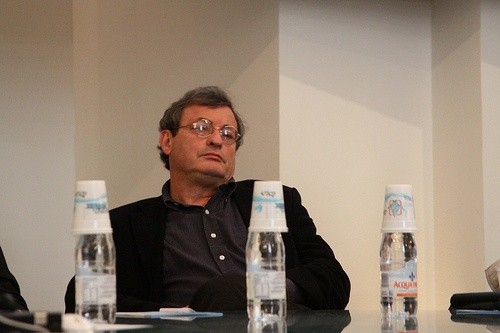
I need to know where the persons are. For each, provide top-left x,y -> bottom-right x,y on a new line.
0,245 -> 34,324
63,86 -> 351,315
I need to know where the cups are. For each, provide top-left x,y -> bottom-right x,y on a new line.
73,180 -> 113,233
380,184 -> 418,233
249,181 -> 288,230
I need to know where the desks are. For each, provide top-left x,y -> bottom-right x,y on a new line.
0,307 -> 500,333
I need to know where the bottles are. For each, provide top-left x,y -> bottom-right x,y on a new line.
75,233 -> 117,324
246,232 -> 287,320
382,316 -> 418,332
381,233 -> 417,317
247,319 -> 287,333
